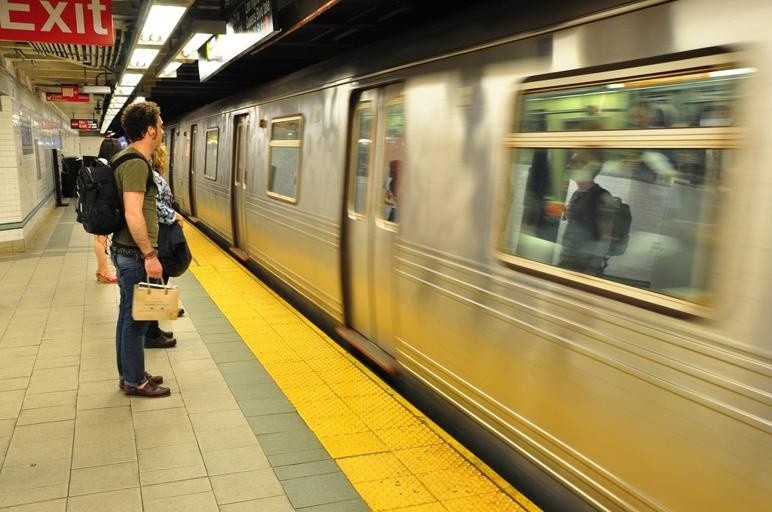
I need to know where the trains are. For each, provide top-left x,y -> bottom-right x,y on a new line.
161,0 -> 771,512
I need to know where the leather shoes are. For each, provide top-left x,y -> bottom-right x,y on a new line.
119,372 -> 171,397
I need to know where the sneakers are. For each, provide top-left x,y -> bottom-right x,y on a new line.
144,327 -> 177,348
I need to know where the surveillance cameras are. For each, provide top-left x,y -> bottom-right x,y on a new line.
95,109 -> 103,116
78,85 -> 112,96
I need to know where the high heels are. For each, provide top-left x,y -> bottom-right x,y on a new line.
96,266 -> 117,284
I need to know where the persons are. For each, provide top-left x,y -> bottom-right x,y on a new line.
90,138 -> 123,284
555,140 -> 616,274
102,100 -> 173,399
620,103 -> 733,188
145,142 -> 184,348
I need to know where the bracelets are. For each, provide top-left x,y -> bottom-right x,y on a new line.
142,250 -> 159,259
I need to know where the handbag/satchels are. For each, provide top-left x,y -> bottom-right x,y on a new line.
157,220 -> 192,276
132,275 -> 179,321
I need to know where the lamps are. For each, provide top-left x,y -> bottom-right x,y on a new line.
105,0 -> 226,119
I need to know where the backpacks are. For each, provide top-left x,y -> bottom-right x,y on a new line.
75,153 -> 153,235
586,188 -> 633,256
